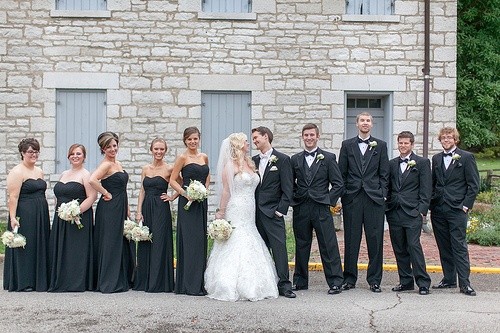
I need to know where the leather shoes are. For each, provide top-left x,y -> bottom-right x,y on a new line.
291,284 -> 308,291
419,286 -> 429,294
431,278 -> 457,289
460,285 -> 476,296
370,284 -> 381,292
327,285 -> 340,294
341,282 -> 355,289
392,284 -> 414,291
278,289 -> 296,298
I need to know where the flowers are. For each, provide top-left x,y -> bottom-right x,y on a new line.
315,153 -> 325,164
268,153 -> 279,167
206,207 -> 237,241
407,159 -> 416,170
55,197 -> 84,230
0,217 -> 27,250
451,153 -> 461,164
368,140 -> 377,151
182,178 -> 208,211
122,216 -> 154,244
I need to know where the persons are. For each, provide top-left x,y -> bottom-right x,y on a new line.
48,144 -> 98,292
289,122 -> 344,294
204,133 -> 279,302
89,131 -> 136,293
4,138 -> 50,292
133,138 -> 183,292
252,126 -> 296,298
386,131 -> 432,294
337,112 -> 389,292
170,127 -> 210,296
431,127 -> 480,296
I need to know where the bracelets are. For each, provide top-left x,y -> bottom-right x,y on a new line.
103,190 -> 107,195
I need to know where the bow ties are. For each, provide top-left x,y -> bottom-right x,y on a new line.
443,148 -> 457,157
304,149 -> 318,156
357,137 -> 370,144
399,157 -> 408,163
